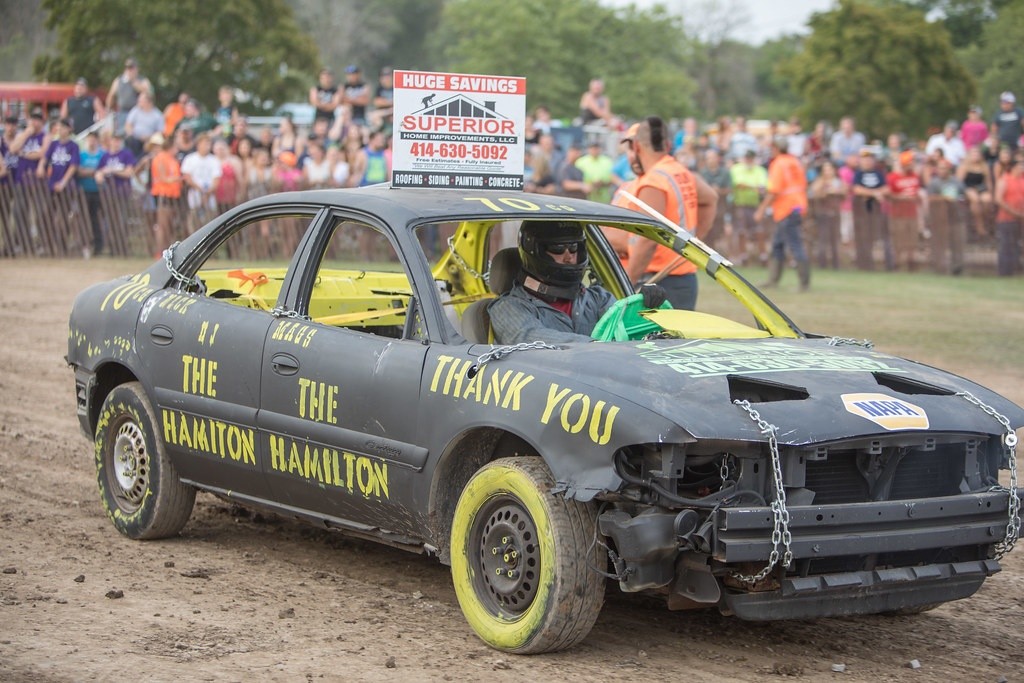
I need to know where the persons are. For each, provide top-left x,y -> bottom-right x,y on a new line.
487,220 -> 617,343
753,141 -> 812,285
0,58 -> 432,263
525,80 -> 636,203
670,91 -> 1024,274
600,124 -> 644,269
625,120 -> 718,311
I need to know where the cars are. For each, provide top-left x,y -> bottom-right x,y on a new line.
61,181 -> 1022,656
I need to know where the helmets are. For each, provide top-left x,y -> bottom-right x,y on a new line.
517,220 -> 589,300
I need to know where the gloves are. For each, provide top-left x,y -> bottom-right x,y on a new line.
639,284 -> 667,308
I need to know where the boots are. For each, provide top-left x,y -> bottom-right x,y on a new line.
758,259 -> 782,288
788,261 -> 810,295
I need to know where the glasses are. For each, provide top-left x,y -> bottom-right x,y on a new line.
544,242 -> 577,254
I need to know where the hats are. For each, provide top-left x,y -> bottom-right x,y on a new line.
900,152 -> 912,165
770,135 -> 787,152
4,105 -> 167,146
968,106 -> 984,117
1001,91 -> 1017,104
620,122 -> 641,144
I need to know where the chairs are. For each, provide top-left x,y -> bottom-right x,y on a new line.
461,246 -> 523,345
